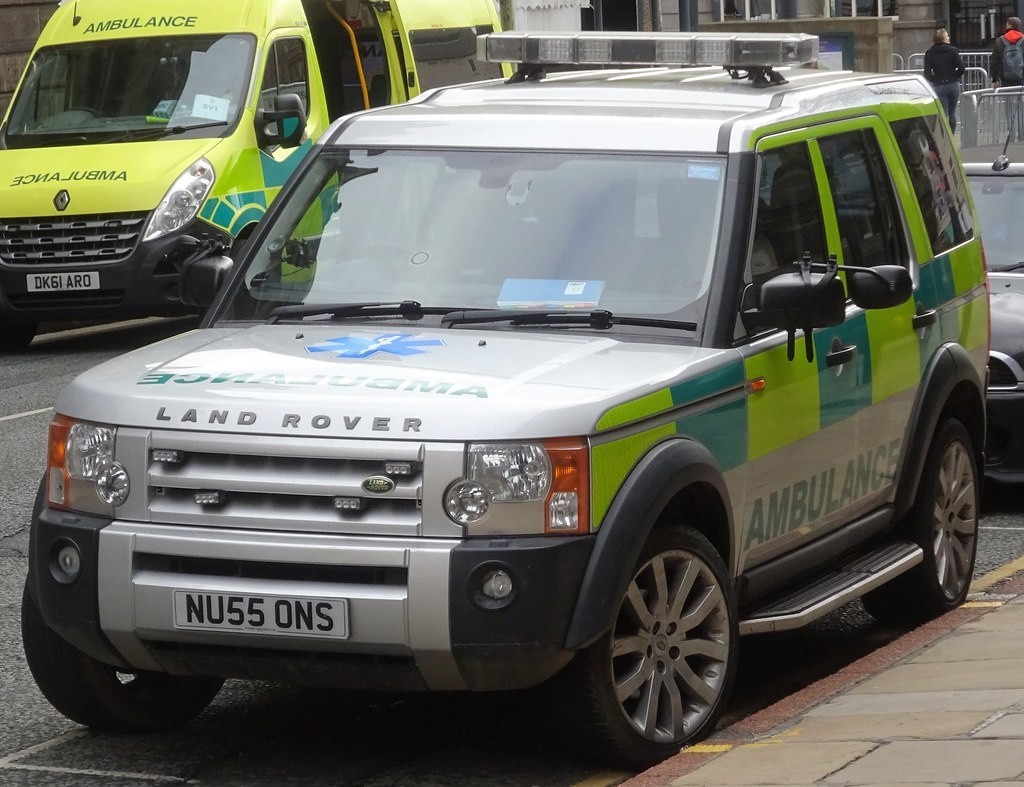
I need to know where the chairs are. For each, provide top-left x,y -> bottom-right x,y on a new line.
658,185 -> 786,270
88,48 -> 161,116
762,163 -> 839,264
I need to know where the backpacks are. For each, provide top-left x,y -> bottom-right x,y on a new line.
1000,34 -> 1023,82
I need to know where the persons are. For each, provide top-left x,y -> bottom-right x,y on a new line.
988,16 -> 1024,142
923,28 -> 967,135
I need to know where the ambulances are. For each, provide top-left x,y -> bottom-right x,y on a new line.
26,34 -> 993,765
1,2 -> 422,352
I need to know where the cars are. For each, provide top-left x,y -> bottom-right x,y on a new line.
962,156 -> 1023,492
